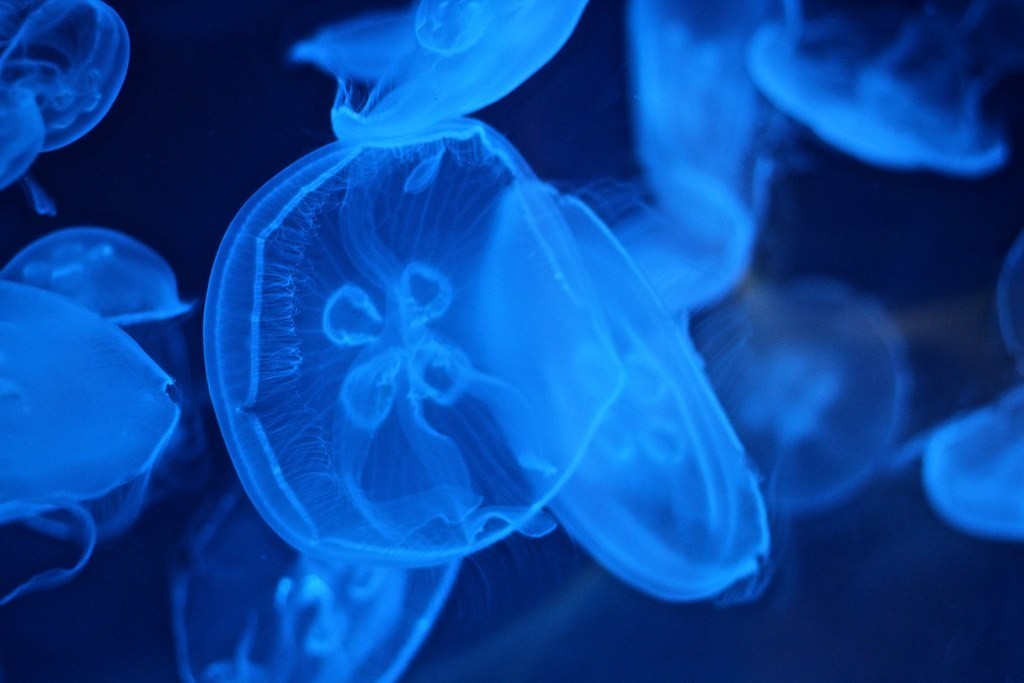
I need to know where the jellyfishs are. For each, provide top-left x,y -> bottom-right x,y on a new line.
0,1 -> 1024,683
